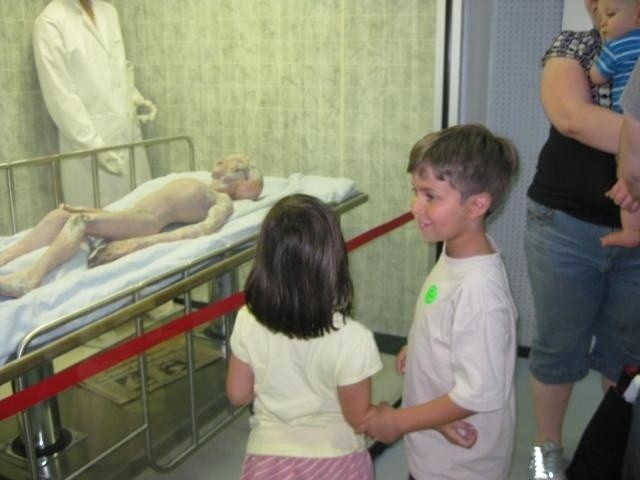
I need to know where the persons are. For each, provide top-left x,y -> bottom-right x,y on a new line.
589,0 -> 640,247
523,0 -> 623,480
33,0 -> 159,211
225,192 -> 478,479
354,124 -> 520,480
564,54 -> 640,479
1,153 -> 266,300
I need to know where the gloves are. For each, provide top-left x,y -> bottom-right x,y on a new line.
137,99 -> 157,125
98,150 -> 127,177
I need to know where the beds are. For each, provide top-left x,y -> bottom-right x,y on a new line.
0,134 -> 367,480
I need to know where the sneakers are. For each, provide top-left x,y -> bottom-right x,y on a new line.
527,441 -> 565,478
